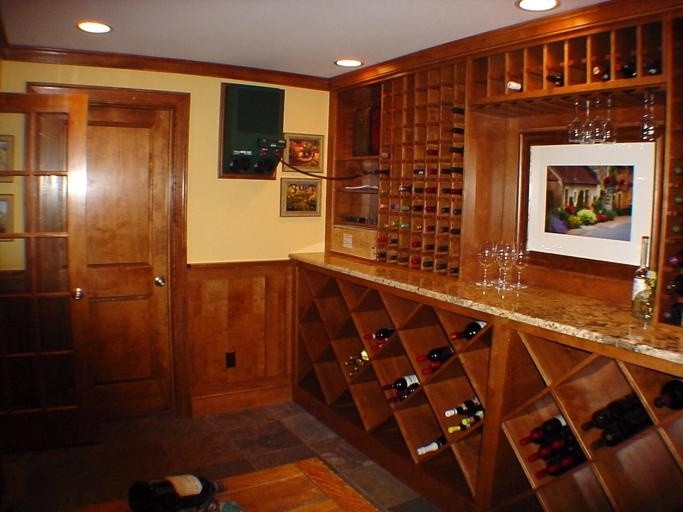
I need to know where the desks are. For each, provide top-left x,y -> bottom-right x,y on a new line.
58,458 -> 379,511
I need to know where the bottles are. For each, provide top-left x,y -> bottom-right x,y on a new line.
417,436 -> 447,455
421,364 -> 440,374
581,393 -> 644,433
630,236 -> 653,313
519,414 -> 587,478
448,422 -> 472,434
457,397 -> 481,414
443,403 -> 475,418
546,73 -> 564,86
450,320 -> 488,341
383,373 -> 418,391
589,411 -> 655,450
386,382 -> 421,405
507,81 -> 522,92
632,270 -> 657,319
653,379 -> 683,411
415,344 -> 455,363
615,62 -> 636,78
591,65 -> 609,80
461,410 -> 484,425
364,326 -> 395,340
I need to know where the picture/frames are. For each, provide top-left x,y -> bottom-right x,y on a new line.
524,140 -> 657,269
0,134 -> 16,183
0,194 -> 16,243
281,179 -> 323,218
282,132 -> 325,174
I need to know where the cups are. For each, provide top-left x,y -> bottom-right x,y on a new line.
588,94 -> 606,142
512,242 -> 528,289
641,91 -> 657,142
601,94 -> 617,143
567,97 -> 583,143
476,240 -> 495,288
580,95 -> 594,145
498,241 -> 512,291
492,247 -> 504,284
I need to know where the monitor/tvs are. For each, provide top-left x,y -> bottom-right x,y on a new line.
225,84 -> 285,152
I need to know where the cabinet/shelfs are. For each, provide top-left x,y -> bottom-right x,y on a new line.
327,2 -> 683,322
290,254 -> 683,512
